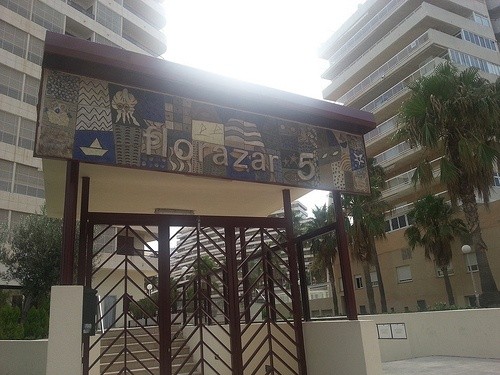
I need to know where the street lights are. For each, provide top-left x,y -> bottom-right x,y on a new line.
461,244 -> 481,308
146,284 -> 153,297
